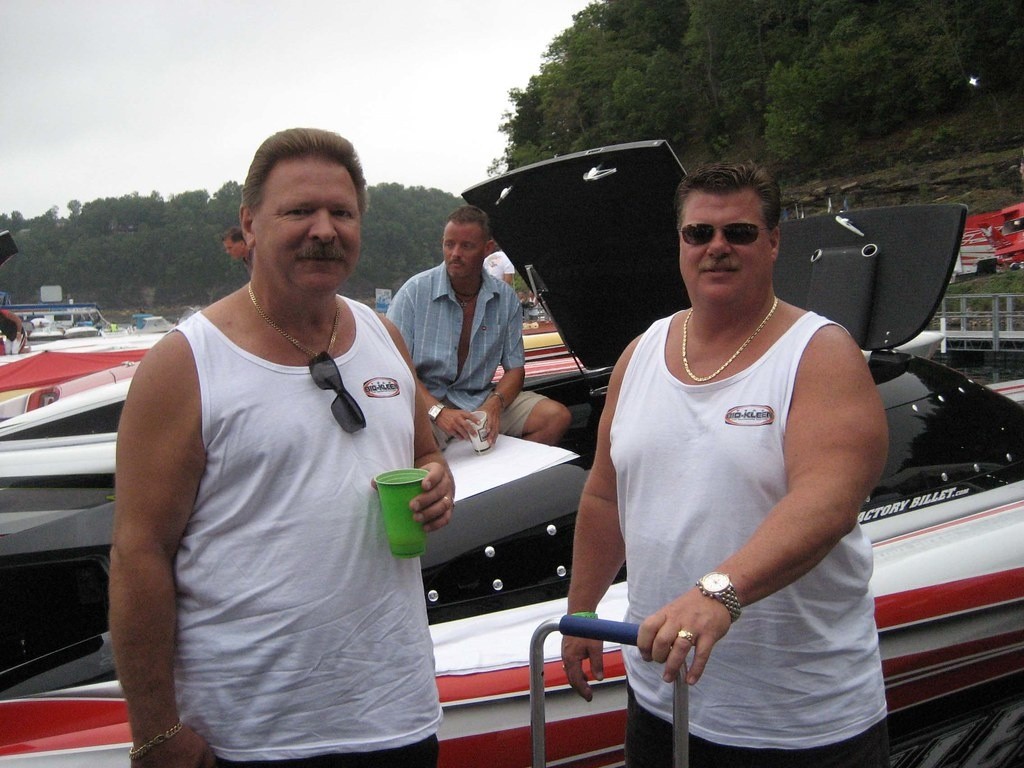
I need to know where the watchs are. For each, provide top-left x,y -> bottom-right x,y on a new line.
427,403 -> 444,424
694,572 -> 741,623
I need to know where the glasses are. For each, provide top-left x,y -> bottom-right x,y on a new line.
678,222 -> 769,245
309,351 -> 366,435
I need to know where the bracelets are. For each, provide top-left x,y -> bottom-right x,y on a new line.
569,612 -> 598,621
489,393 -> 505,411
125,721 -> 183,759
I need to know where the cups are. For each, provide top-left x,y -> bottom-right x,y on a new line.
465,411 -> 492,456
373,468 -> 431,559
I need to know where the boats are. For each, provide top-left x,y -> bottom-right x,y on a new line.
0,229 -> 1024,768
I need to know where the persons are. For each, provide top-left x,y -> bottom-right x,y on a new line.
0,310 -> 23,356
560,161 -> 896,768
110,130 -> 580,768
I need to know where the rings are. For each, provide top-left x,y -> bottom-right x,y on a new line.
444,495 -> 454,505
678,631 -> 694,646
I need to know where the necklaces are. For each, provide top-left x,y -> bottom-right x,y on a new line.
451,286 -> 480,307
247,281 -> 341,366
681,295 -> 778,382
